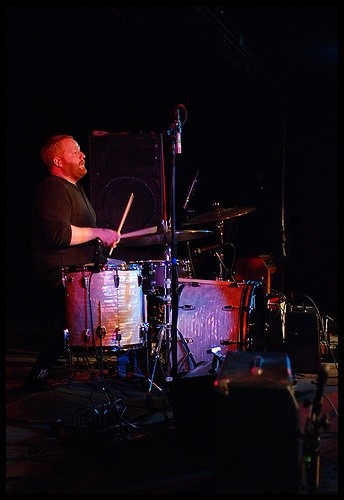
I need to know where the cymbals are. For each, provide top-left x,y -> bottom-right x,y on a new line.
121,230 -> 214,245
182,207 -> 255,226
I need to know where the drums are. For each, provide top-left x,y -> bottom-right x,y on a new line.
59,265 -> 143,350
129,260 -> 192,296
166,280 -> 265,377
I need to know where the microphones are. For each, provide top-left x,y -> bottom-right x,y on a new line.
193,244 -> 221,255
93,131 -> 127,137
174,109 -> 183,154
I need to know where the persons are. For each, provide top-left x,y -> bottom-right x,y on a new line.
33,134 -> 177,382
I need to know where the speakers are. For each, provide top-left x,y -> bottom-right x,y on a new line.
164,372 -> 301,462
267,310 -> 323,373
88,134 -> 168,237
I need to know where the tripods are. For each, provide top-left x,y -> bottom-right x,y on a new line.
145,266 -> 196,396
